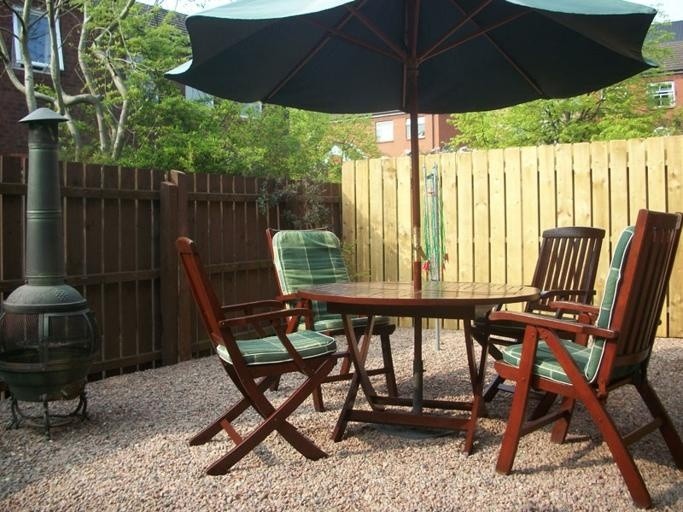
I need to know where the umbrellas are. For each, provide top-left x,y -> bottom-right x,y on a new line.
160,1 -> 656,280
